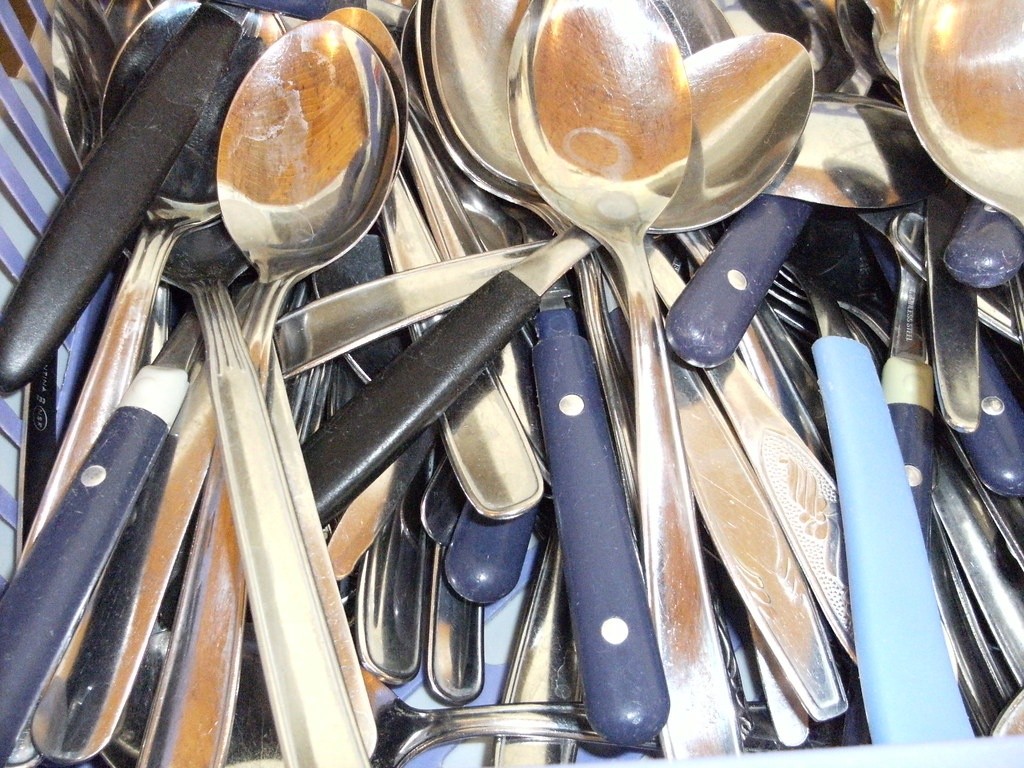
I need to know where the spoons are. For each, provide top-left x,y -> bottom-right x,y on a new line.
0,0 -> 1024,767
